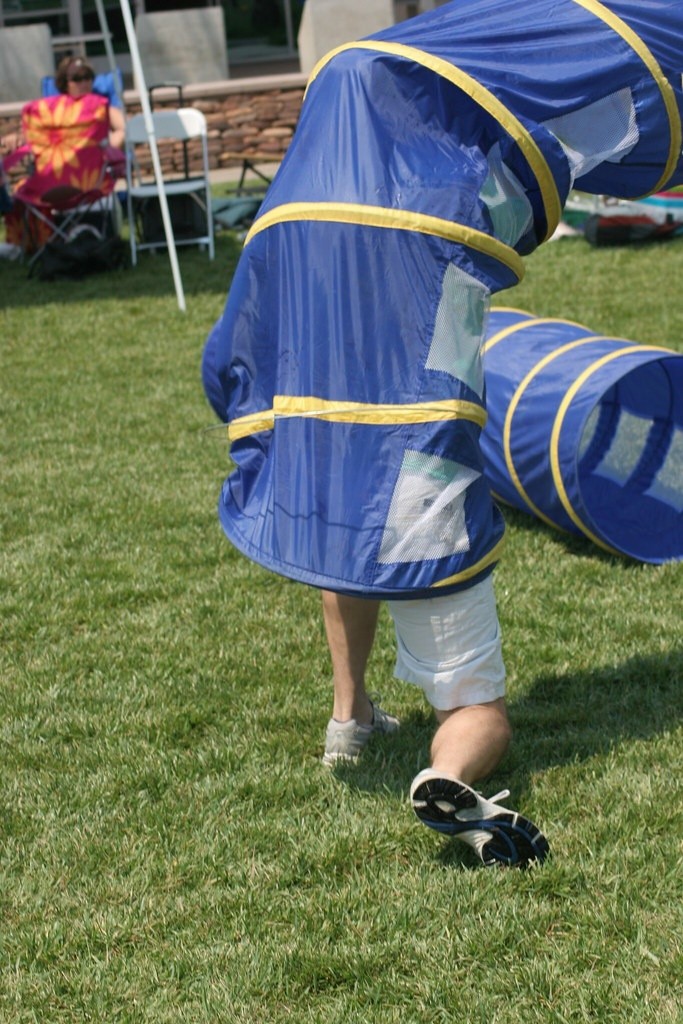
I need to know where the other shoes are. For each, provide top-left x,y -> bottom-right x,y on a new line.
0,243 -> 21,261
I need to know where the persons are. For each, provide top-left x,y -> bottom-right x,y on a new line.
212,45 -> 552,869
0,55 -> 125,281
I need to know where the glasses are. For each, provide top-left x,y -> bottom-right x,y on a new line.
70,71 -> 94,83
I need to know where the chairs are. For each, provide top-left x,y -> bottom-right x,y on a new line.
124,108 -> 214,266
40,70 -> 123,111
3,94 -> 123,281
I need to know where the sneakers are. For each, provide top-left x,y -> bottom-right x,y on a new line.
321,698 -> 399,767
410,767 -> 552,872
